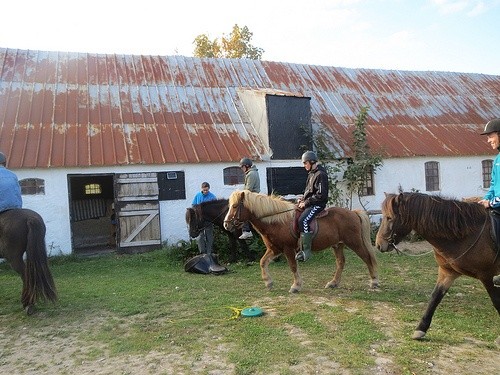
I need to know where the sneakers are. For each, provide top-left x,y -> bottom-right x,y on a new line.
239,231 -> 254,239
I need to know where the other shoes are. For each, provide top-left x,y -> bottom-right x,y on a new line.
493,275 -> 500,284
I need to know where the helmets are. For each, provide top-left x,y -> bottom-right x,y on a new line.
302,151 -> 318,162
481,118 -> 500,135
240,158 -> 252,166
0,152 -> 7,164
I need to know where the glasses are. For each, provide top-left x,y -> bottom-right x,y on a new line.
241,164 -> 246,167
303,161 -> 310,165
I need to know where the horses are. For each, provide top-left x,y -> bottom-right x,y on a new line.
222,189 -> 380,293
185,197 -> 261,261
374,191 -> 500,340
0,209 -> 59,317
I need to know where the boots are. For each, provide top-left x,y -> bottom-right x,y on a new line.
296,233 -> 312,261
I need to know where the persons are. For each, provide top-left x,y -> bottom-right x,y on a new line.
0,152 -> 23,214
239,158 -> 260,239
295,151 -> 328,261
192,182 -> 217,254
478,118 -> 500,287
